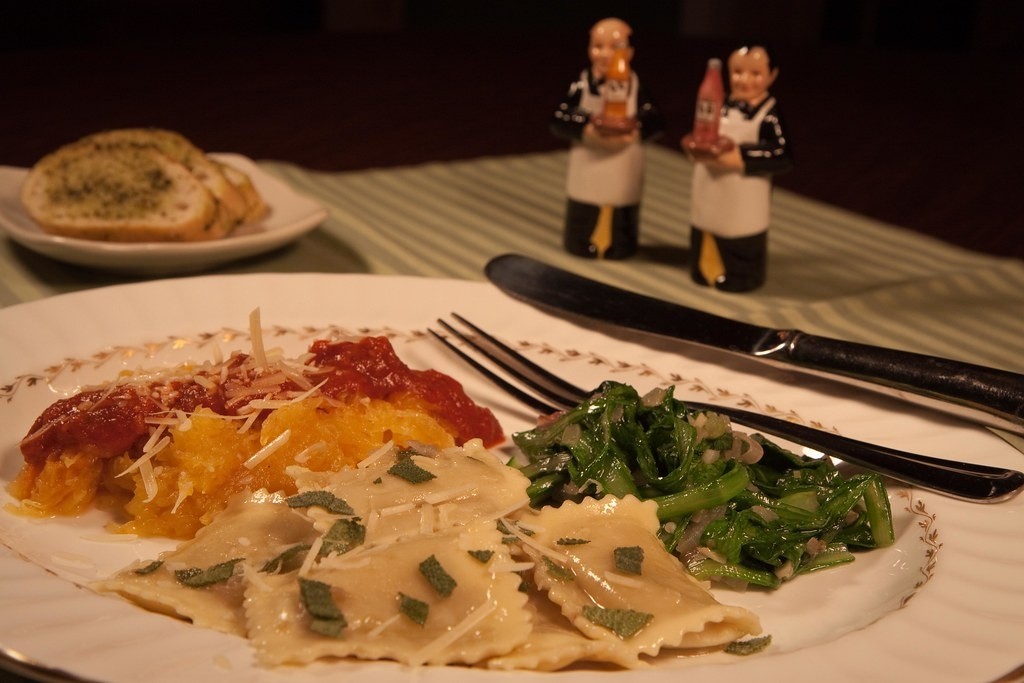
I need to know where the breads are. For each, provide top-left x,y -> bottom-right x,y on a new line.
22,129 -> 260,241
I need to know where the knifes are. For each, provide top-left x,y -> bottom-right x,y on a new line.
484,252 -> 1024,421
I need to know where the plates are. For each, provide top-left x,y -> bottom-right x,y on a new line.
0,151 -> 326,276
0,272 -> 1024,683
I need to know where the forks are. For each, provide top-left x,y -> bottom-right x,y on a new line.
425,310 -> 1024,505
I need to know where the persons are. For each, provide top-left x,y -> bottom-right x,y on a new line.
550,17 -> 659,263
681,38 -> 793,294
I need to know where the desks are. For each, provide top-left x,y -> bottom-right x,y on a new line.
0,0 -> 1024,683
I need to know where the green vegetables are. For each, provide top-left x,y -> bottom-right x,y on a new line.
505,380 -> 894,585
